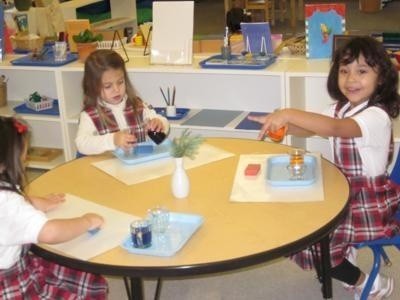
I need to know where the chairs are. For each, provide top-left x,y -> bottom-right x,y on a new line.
353,146 -> 400,300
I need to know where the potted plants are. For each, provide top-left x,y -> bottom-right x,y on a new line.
170,129 -> 203,199
72,30 -> 102,64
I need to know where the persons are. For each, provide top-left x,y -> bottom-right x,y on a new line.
227,6 -> 252,45
75,48 -> 170,157
246,36 -> 400,300
0,114 -> 108,300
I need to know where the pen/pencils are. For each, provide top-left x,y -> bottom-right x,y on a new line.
172,86 -> 175,105
160,86 -> 170,106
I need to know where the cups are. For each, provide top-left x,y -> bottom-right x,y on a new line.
147,207 -> 171,247
53,42 -> 67,61
129,219 -> 153,248
134,36 -> 142,46
290,147 -> 304,177
123,129 -> 139,157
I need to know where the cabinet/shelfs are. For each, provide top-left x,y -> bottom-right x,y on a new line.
0,55 -> 68,170
59,0 -> 137,37
285,60 -> 400,161
57,58 -> 296,162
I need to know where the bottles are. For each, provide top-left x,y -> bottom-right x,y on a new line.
269,124 -> 285,142
141,118 -> 167,146
221,27 -> 231,60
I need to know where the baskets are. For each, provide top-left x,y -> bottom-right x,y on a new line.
10,31 -> 47,53
25,97 -> 54,112
97,37 -> 127,50
287,42 -> 305,55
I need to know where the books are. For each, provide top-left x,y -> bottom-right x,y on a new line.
305,4 -> 346,58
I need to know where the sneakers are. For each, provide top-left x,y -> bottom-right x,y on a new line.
354,274 -> 395,300
342,247 -> 359,290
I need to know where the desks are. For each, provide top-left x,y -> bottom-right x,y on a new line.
19,138 -> 351,300
